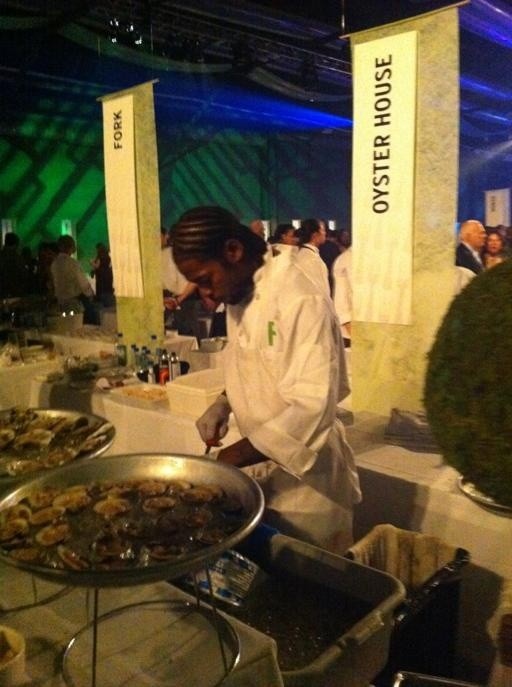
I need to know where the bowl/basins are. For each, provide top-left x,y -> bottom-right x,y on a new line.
0,625 -> 27,686
198,338 -> 224,353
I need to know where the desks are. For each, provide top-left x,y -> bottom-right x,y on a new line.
0,564 -> 283,686
0,285 -> 512,582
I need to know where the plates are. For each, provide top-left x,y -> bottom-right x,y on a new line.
457,472 -> 511,513
2,449 -> 268,587
2,405 -> 115,483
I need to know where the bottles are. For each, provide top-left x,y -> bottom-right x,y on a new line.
115,332 -> 181,385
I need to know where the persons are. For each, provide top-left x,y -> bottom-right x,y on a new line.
170,205 -> 365,561
162,220 -> 351,347
455,219 -> 509,272
2,233 -> 113,320
